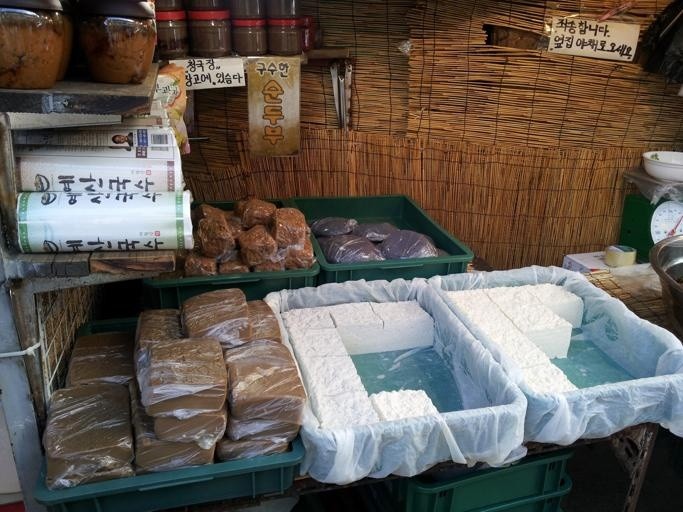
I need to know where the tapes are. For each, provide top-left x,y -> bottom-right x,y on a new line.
605,245 -> 637,267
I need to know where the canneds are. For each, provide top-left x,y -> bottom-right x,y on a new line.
0,0 -> 315,89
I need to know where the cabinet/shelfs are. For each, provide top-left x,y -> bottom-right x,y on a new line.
2,50 -> 309,296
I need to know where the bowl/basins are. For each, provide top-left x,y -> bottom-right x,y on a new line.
648,234 -> 683,324
642,151 -> 683,183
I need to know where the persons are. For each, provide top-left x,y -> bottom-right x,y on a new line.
112,132 -> 133,147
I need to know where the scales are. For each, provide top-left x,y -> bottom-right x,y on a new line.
618,169 -> 683,263
